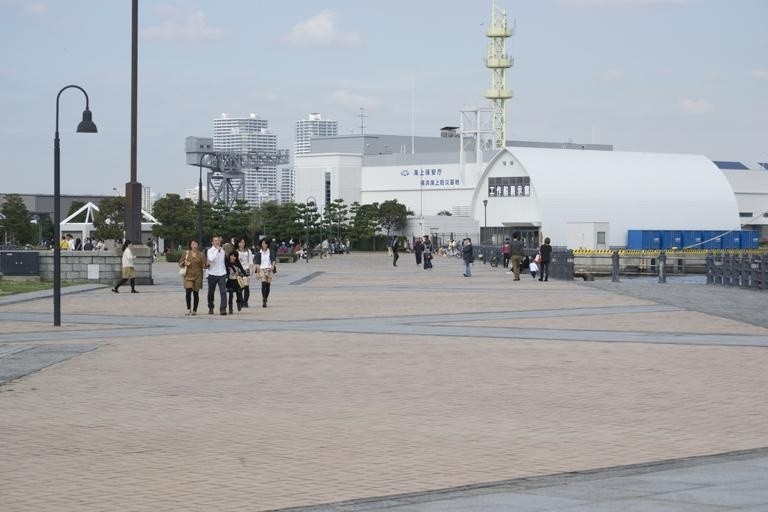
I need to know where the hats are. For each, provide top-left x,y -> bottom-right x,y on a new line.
423,235 -> 428,238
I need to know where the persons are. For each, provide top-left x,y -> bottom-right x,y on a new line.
44,234 -> 104,250
502,238 -> 542,278
509,233 -> 525,281
177,235 -> 277,316
267,236 -> 352,263
147,237 -> 158,261
111,239 -> 139,294
386,234 -> 475,277
537,237 -> 553,283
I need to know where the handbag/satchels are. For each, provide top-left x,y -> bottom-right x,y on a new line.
424,253 -> 432,260
237,265 -> 250,288
273,265 -> 276,273
178,265 -> 187,276
535,251 -> 542,263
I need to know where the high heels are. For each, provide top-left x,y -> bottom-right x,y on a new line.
112,288 -> 119,293
131,290 -> 139,293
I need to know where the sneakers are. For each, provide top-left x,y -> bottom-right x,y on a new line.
192,310 -> 197,315
185,309 -> 191,316
209,307 -> 213,314
237,305 -> 241,311
220,308 -> 227,315
228,307 -> 233,314
262,300 -> 266,307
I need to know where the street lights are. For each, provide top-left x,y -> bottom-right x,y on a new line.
197,149 -> 224,248
483,199 -> 490,241
51,81 -> 99,327
304,194 -> 318,263
112,187 -> 121,196
